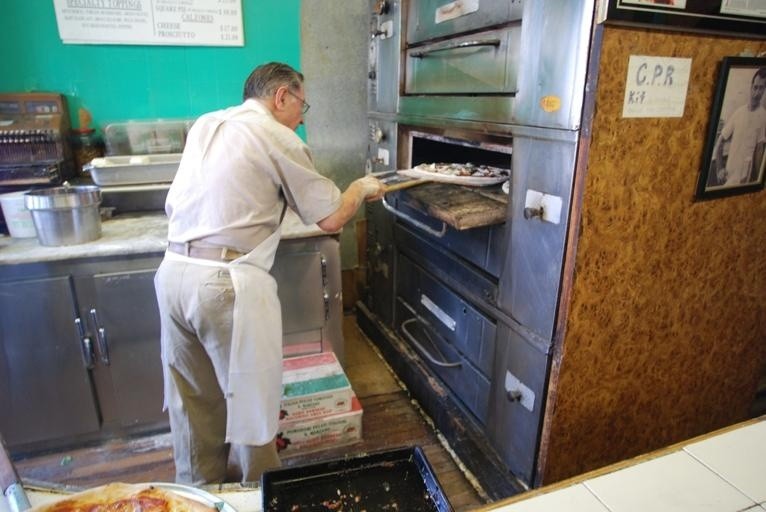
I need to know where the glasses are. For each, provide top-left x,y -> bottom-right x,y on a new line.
288,90 -> 311,115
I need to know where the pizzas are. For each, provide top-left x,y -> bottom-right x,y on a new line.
37,481 -> 219,512
415,161 -> 508,184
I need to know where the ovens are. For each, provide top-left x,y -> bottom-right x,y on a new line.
364,1 -> 611,492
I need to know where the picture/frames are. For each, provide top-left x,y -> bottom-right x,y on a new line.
597,0 -> 766,39
695,55 -> 766,201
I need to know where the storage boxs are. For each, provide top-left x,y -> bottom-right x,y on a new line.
267,352 -> 352,423
275,388 -> 364,459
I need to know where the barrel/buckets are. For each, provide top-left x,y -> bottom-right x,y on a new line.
0,192 -> 36,239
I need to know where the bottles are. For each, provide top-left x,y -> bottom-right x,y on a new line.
72,124 -> 101,177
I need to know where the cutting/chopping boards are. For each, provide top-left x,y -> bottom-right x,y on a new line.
396,161 -> 511,186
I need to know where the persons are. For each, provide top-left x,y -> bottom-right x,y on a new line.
152,59 -> 388,486
713,68 -> 765,188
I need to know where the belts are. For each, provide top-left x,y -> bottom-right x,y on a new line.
169,240 -> 247,264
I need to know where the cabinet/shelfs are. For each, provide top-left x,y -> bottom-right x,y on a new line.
0,233 -> 343,461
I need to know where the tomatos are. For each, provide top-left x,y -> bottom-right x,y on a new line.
280,409 -> 288,420
277,433 -> 292,451
